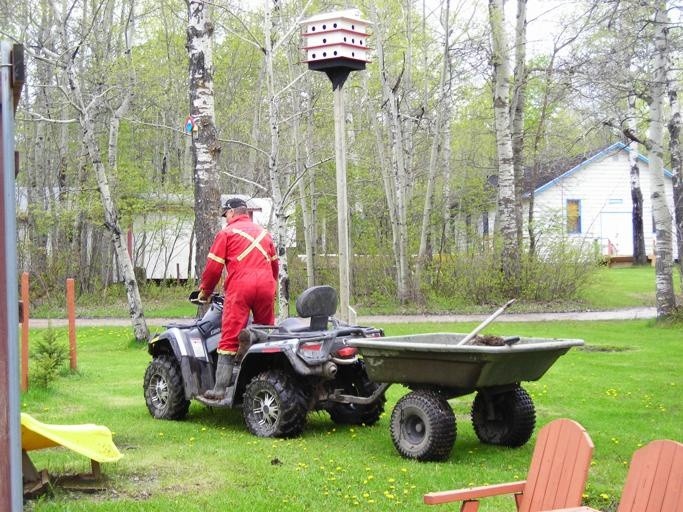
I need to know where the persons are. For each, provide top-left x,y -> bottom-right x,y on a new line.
197,198 -> 279,400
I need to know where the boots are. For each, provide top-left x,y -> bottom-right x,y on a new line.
204,353 -> 235,400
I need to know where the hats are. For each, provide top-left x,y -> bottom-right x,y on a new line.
221,198 -> 247,217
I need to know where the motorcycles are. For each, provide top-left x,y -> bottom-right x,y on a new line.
134,283 -> 392,443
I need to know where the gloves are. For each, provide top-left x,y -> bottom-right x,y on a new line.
198,289 -> 212,304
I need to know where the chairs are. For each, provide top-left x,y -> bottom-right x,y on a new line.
615,439 -> 683,512
426,418 -> 594,511
269,285 -> 338,333
